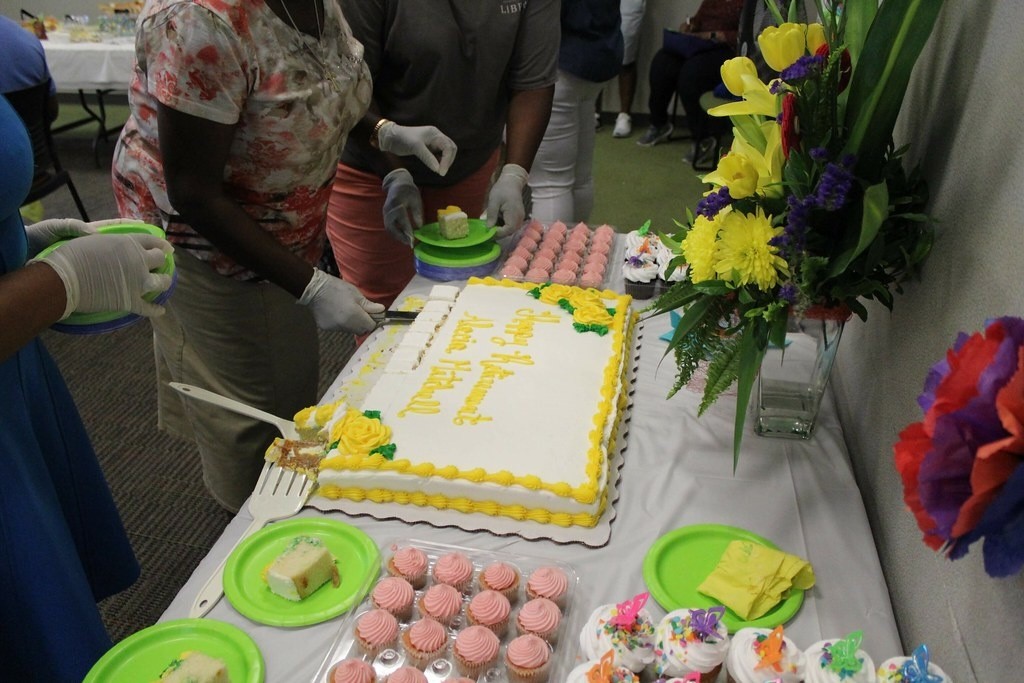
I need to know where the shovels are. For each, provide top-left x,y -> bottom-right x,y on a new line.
187,461 -> 318,621
167,381 -> 324,457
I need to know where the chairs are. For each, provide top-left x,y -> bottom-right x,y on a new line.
669,0 -> 758,172
2,76 -> 93,225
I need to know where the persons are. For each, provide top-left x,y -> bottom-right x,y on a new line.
0,93 -> 175,683
594,0 -> 647,137
638,0 -> 757,163
502,0 -> 626,229
326,0 -> 560,347
715,0 -> 819,101
111,0 -> 457,512
0,15 -> 58,175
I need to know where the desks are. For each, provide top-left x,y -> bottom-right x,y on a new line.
31,30 -> 141,168
158,218 -> 910,683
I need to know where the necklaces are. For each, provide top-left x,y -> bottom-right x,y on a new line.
280,0 -> 340,92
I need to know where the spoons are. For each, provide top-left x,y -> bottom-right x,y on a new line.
169,381 -> 302,440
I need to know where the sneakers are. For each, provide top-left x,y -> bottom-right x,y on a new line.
636,123 -> 672,148
681,138 -> 716,165
612,113 -> 632,137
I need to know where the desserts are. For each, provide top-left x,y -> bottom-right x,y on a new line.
327,545 -> 568,683
566,591 -> 952,683
500,220 -> 688,300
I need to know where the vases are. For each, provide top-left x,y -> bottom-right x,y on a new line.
755,300 -> 854,442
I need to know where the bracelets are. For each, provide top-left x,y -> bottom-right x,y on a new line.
710,31 -> 716,39
369,118 -> 388,148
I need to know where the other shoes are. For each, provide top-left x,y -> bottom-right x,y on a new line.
595,113 -> 605,130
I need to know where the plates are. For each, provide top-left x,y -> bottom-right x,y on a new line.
641,526 -> 805,633
83,619 -> 265,683
413,221 -> 500,280
224,520 -> 380,627
30,222 -> 177,334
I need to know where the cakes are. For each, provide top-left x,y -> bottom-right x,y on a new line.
292,276 -> 634,528
437,206 -> 468,239
159,649 -> 227,683
267,541 -> 336,600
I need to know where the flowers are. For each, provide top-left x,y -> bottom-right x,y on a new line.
683,29 -> 932,342
893,313 -> 1024,585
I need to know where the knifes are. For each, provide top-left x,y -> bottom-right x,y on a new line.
368,309 -> 418,321
